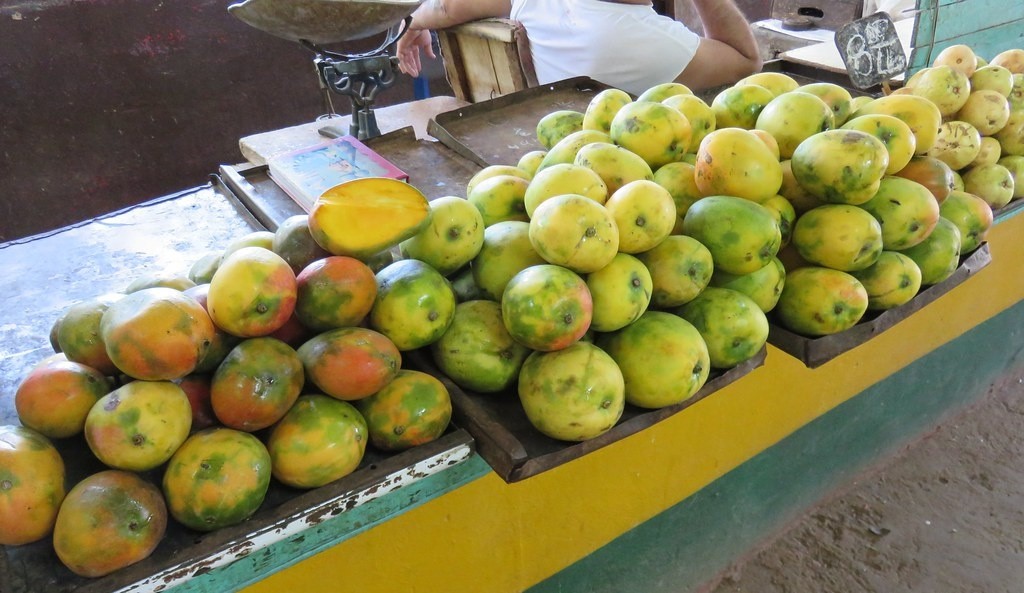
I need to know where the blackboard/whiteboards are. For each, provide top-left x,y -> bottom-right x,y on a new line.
835,11 -> 907,88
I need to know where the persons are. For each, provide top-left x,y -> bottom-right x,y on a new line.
395,0 -> 763,97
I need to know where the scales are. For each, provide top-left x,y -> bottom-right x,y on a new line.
228,1 -> 428,142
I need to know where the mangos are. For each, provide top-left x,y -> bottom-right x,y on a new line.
0,175 -> 451,577
416,42 -> 1023,441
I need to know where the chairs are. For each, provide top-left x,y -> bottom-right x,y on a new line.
432,17 -> 539,102
